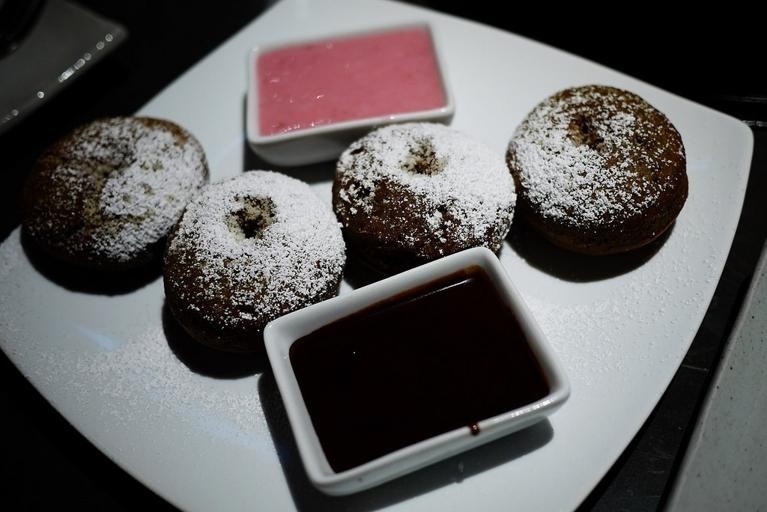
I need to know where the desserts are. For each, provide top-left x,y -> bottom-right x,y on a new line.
25,84 -> 686,351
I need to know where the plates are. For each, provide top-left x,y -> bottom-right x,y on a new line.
262,245 -> 573,498
1,1 -> 755,512
245,19 -> 450,172
